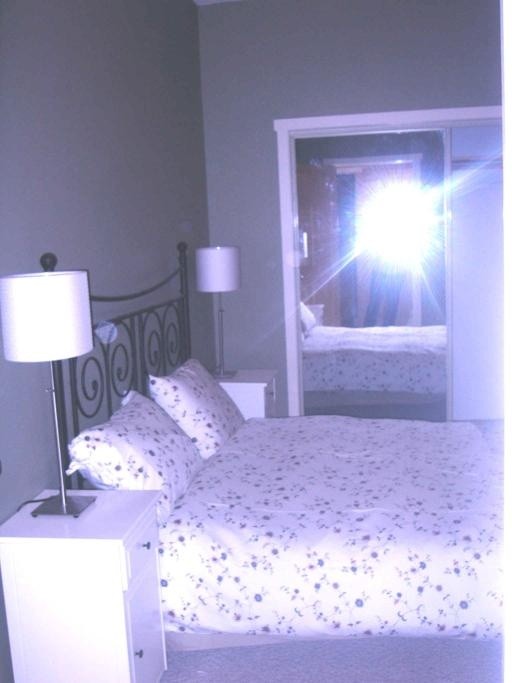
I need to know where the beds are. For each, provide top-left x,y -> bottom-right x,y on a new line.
39,241 -> 502,654
299,300 -> 449,419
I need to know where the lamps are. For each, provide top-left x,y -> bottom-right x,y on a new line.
195,246 -> 241,379
0,269 -> 96,519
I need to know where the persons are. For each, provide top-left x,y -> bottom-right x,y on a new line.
364,275 -> 401,326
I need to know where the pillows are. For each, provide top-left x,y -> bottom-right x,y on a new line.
299,300 -> 318,335
66,356 -> 244,527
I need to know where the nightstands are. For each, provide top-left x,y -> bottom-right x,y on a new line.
211,367 -> 279,420
0,488 -> 166,683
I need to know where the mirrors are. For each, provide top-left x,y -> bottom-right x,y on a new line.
291,126 -> 454,422
